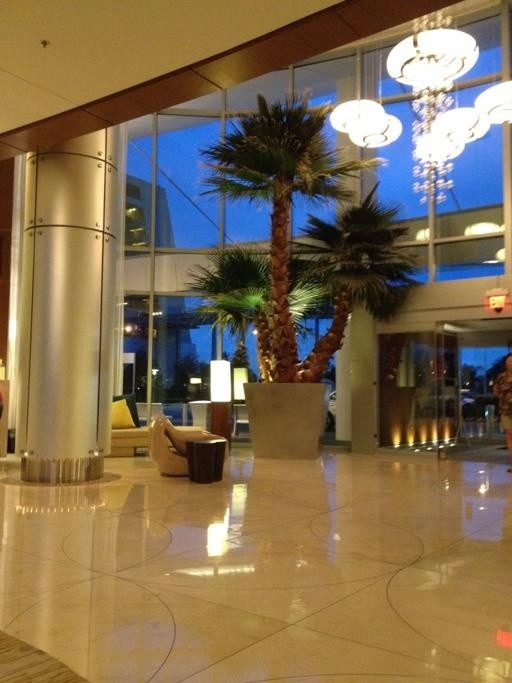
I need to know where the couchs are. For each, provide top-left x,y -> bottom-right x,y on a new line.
105,425 -> 205,457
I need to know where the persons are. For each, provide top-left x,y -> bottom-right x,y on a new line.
492,352 -> 512,473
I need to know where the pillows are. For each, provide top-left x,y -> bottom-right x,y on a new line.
113,394 -> 138,427
111,399 -> 136,428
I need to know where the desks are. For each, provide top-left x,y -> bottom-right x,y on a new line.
188,400 -> 210,432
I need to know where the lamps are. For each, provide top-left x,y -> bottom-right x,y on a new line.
415,221 -> 505,264
209,359 -> 231,454
205,504 -> 231,556
328,0 -> 512,206
233,366 -> 248,404
487,283 -> 510,314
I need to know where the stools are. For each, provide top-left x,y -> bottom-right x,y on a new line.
183,438 -> 227,483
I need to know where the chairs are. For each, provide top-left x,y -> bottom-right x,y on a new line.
147,414 -> 230,477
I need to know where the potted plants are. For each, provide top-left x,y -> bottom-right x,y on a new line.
172,84 -> 414,460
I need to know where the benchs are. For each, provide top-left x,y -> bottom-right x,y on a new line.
232,404 -> 250,436
136,403 -> 175,421
